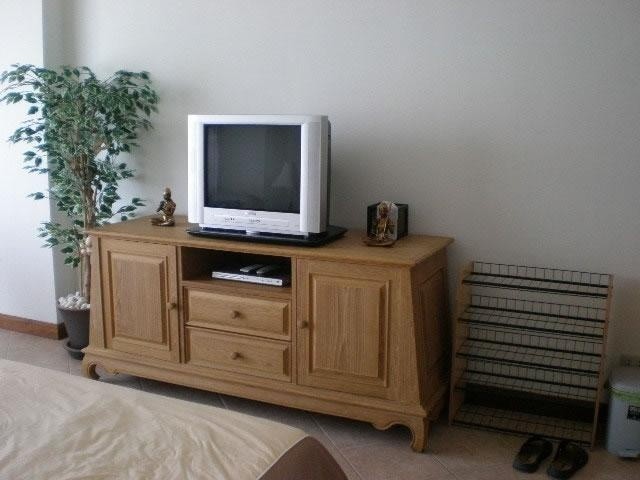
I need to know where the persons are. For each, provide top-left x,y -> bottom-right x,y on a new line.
149,188 -> 177,226
367,202 -> 395,240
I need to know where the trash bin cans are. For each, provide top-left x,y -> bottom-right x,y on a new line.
605,367 -> 640,458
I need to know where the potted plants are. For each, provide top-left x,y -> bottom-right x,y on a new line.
1,64 -> 161,349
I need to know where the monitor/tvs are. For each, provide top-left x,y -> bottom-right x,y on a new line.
188,115 -> 331,238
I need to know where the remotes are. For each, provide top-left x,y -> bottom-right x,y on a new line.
256,263 -> 276,275
240,263 -> 266,274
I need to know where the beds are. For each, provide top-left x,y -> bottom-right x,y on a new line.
1,359 -> 346,479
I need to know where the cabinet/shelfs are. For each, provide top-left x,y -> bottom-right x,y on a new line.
448,259 -> 614,449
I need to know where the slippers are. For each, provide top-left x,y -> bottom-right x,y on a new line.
512,437 -> 553,473
546,440 -> 589,479
